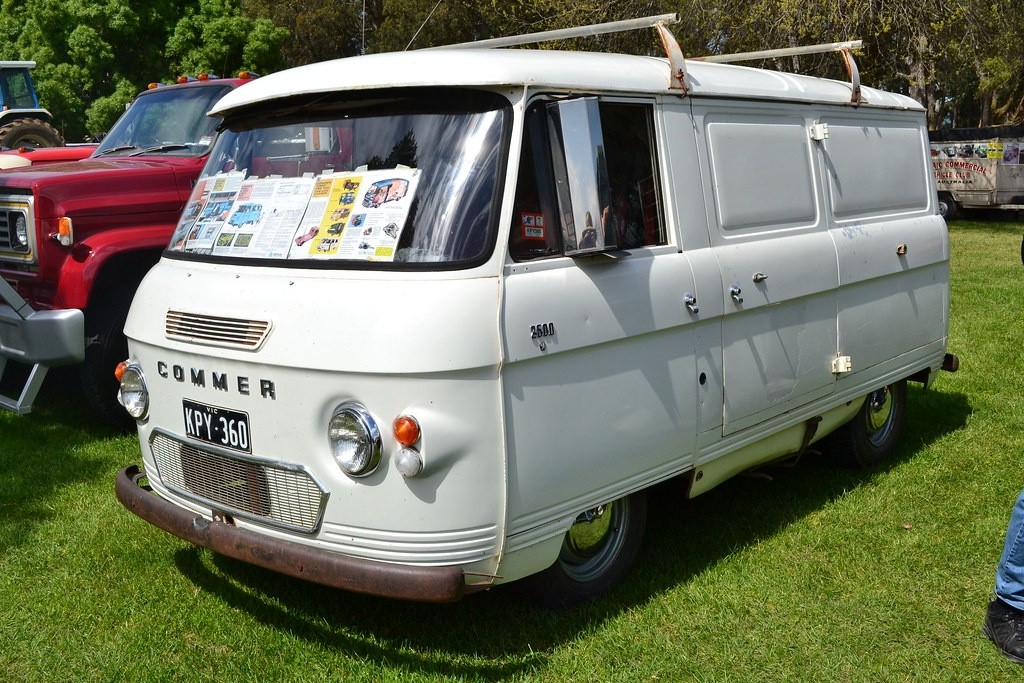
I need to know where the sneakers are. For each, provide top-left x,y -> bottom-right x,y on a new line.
983,597 -> 1024,664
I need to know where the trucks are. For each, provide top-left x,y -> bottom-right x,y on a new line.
0,71 -> 359,426
927,127 -> 1024,218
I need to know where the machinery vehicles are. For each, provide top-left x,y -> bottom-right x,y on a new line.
0,60 -> 66,150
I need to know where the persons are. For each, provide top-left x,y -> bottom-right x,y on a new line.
579,212 -> 597,249
981,234 -> 1024,663
596,145 -> 616,246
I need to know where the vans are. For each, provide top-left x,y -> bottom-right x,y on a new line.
116,13 -> 962,601
